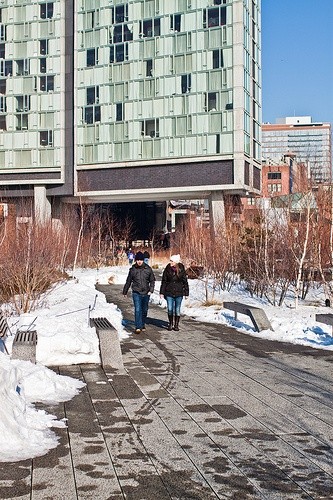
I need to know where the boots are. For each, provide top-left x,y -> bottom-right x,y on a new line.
168,314 -> 173,331
174,315 -> 180,331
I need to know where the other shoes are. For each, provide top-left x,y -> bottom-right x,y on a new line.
136,329 -> 141,334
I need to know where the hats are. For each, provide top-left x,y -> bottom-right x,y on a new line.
135,251 -> 144,261
129,248 -> 131,250
170,254 -> 180,263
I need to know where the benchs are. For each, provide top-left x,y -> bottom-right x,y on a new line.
89,317 -> 123,369
224,301 -> 274,334
0,309 -> 37,364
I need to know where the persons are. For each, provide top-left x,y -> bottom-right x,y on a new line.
122,252 -> 156,333
128,251 -> 134,266
159,254 -> 189,331
144,250 -> 150,264
